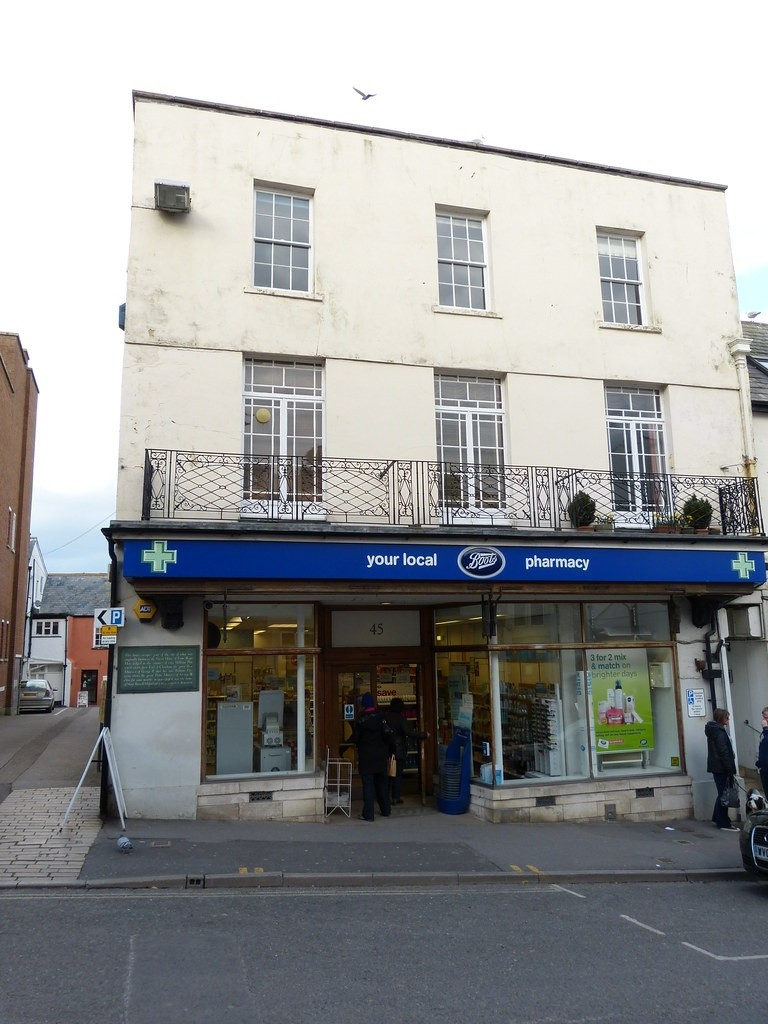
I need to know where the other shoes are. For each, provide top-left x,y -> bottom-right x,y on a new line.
711,821 -> 740,832
358,814 -> 374,821
392,799 -> 404,806
379,810 -> 390,817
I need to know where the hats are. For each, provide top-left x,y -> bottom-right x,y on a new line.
360,692 -> 375,708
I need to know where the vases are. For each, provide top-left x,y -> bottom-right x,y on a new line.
593,524 -> 613,534
654,526 -> 695,534
708,528 -> 720,535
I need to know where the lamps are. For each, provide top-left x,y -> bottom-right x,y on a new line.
154,179 -> 191,216
160,594 -> 184,630
691,598 -> 709,628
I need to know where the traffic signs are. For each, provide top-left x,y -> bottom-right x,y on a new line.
93,607 -> 126,629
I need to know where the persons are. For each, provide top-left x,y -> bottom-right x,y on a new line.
339,678 -> 363,758
387,698 -> 430,805
704,709 -> 741,832
353,692 -> 393,820
755,706 -> 768,801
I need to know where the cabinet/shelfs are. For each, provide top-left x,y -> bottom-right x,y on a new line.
207,695 -> 227,773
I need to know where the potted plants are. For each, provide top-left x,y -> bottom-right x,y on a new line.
692,498 -> 714,536
568,491 -> 596,532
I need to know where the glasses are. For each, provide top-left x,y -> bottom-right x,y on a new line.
725,717 -> 729,720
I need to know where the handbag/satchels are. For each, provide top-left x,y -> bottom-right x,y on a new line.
720,776 -> 740,808
388,754 -> 396,777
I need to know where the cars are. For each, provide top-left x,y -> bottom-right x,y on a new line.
18,679 -> 58,714
737,806 -> 768,876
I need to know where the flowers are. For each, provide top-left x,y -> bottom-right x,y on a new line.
653,511 -> 694,525
596,512 -> 619,522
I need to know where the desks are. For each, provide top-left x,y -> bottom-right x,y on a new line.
468,686 -> 536,779
